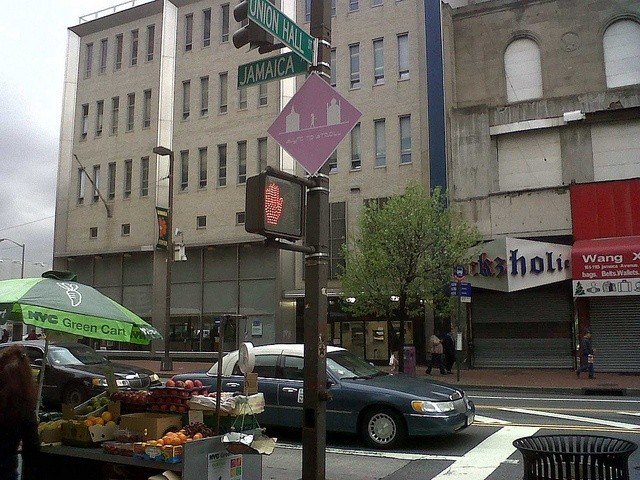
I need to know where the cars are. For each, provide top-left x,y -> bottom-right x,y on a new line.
171,344 -> 475,450
0,340 -> 162,411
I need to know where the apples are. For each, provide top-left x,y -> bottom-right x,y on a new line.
112,391 -> 150,404
151,379 -> 211,413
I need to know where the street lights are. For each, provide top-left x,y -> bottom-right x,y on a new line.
0,238 -> 25,278
152,146 -> 174,372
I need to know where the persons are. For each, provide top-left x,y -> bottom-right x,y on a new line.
424,329 -> 448,377
389,340 -> 399,371
2,329 -> 9,343
25,329 -> 39,340
444,328 -> 456,375
574,329 -> 596,379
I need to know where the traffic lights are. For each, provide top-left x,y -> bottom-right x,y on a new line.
245,175 -> 305,240
232,0 -> 274,54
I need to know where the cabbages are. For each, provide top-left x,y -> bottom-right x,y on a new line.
86,396 -> 111,410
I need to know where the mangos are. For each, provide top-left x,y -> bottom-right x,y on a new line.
84,411 -> 116,428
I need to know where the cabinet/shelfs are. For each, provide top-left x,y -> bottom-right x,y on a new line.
38,390 -> 264,480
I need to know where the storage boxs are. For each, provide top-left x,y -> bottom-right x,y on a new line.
190,394 -> 265,431
61,422 -> 119,448
121,412 -> 182,441
74,390 -> 110,420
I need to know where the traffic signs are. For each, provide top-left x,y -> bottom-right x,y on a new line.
248,0 -> 318,66
450,282 -> 472,303
237,52 -> 311,89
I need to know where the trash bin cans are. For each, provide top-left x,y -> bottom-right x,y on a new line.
513,434 -> 639,480
404,347 -> 415,377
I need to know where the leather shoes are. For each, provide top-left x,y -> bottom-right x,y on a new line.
575,370 -> 580,378
425,371 -> 432,376
447,371 -> 454,374
588,375 -> 596,379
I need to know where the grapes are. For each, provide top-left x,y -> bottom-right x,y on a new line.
183,421 -> 216,438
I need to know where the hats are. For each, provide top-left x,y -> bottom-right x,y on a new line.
584,331 -> 591,332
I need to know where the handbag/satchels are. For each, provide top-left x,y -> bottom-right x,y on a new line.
588,354 -> 593,363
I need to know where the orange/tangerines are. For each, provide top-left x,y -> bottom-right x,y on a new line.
198,433 -> 203,439
194,435 -> 199,439
157,432 -> 187,446
